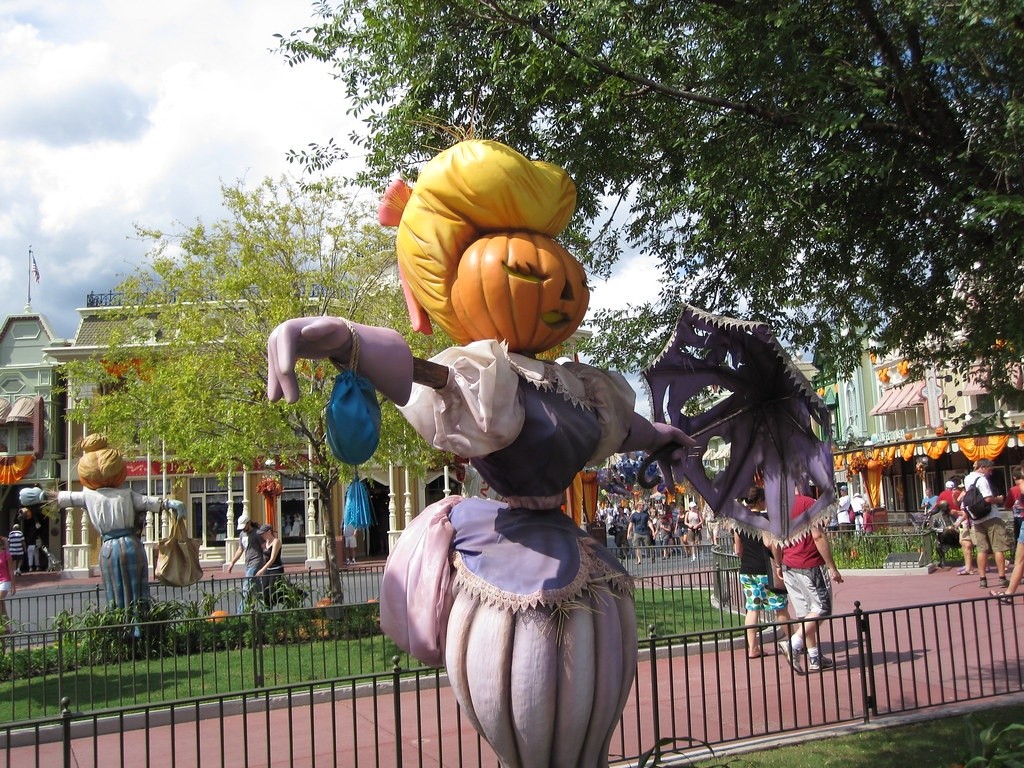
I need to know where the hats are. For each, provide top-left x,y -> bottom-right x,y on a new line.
839,485 -> 848,491
945,481 -> 955,488
688,501 -> 697,507
256,525 -> 270,534
237,515 -> 249,530
13,524 -> 19,529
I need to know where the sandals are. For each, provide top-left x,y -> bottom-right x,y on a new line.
989,590 -> 1013,604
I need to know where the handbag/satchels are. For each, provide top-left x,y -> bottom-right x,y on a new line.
848,504 -> 855,522
154,510 -> 204,587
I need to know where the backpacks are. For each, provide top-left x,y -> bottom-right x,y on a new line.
963,477 -> 991,521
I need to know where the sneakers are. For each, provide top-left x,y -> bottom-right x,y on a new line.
809,654 -> 833,670
777,640 -> 803,673
978,580 -> 987,588
998,579 -> 1009,588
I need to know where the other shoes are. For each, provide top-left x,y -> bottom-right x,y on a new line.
352,558 -> 355,563
29,568 -> 33,571
14,570 -> 21,576
346,558 -> 350,565
36,567 -> 42,571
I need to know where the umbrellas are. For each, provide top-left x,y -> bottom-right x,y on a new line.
638,303 -> 839,548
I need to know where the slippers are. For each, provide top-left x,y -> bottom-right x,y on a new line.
749,651 -> 769,659
957,569 -> 974,575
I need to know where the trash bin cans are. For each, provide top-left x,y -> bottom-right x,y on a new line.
588,521 -> 608,549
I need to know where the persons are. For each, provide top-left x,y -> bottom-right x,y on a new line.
0,536 -> 16,632
19,433 -> 187,637
837,486 -> 851,524
733,484 -> 842,675
289,513 -> 304,536
7,518 -> 42,575
851,493 -> 865,535
229,514 -> 284,615
921,458 -> 1024,604
344,524 -> 357,563
610,501 -> 718,565
267,140 -> 696,768
